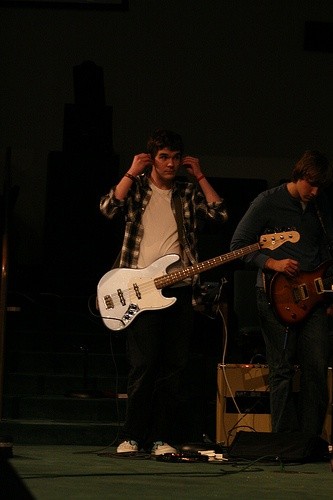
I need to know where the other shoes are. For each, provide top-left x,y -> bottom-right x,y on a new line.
117,440 -> 138,453
154,443 -> 176,456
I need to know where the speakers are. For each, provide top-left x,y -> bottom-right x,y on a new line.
228,430 -> 333,462
234,271 -> 260,330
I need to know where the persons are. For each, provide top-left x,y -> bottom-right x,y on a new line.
99,133 -> 230,459
230,150 -> 333,465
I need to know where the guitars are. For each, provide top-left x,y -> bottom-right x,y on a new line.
268,260 -> 333,324
97,227 -> 300,331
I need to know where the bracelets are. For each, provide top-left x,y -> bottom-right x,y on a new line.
197,174 -> 205,180
126,173 -> 135,180
